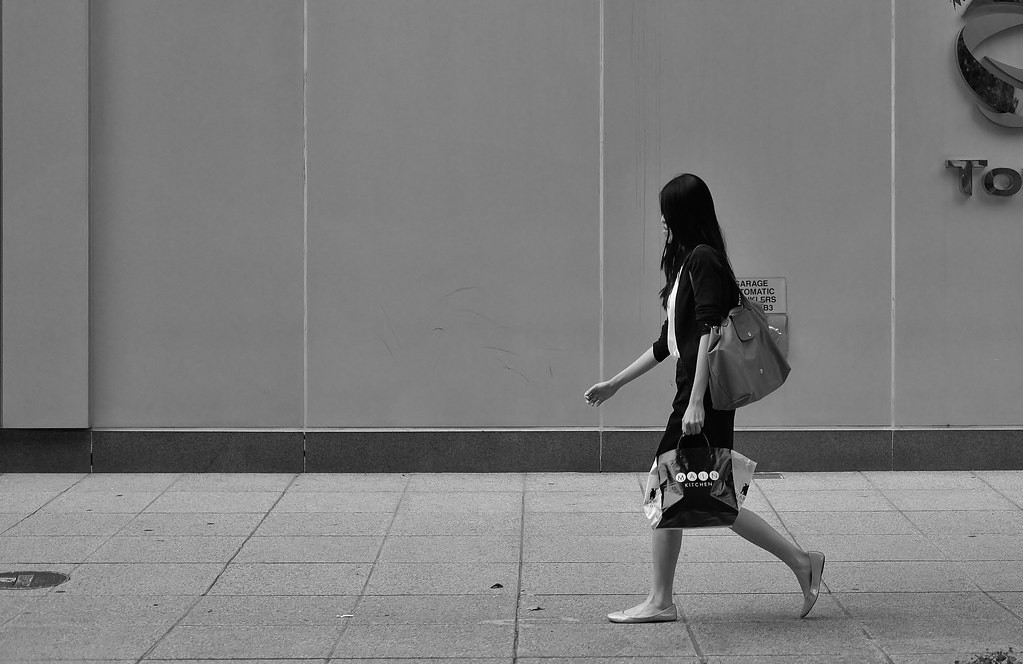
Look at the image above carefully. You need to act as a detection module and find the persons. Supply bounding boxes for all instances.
[581,174,827,623]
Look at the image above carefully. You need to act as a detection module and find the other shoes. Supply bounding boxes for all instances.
[606,603,677,622]
[800,551,825,618]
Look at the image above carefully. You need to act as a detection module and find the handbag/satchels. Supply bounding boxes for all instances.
[689,244,791,410]
[643,431,757,531]
